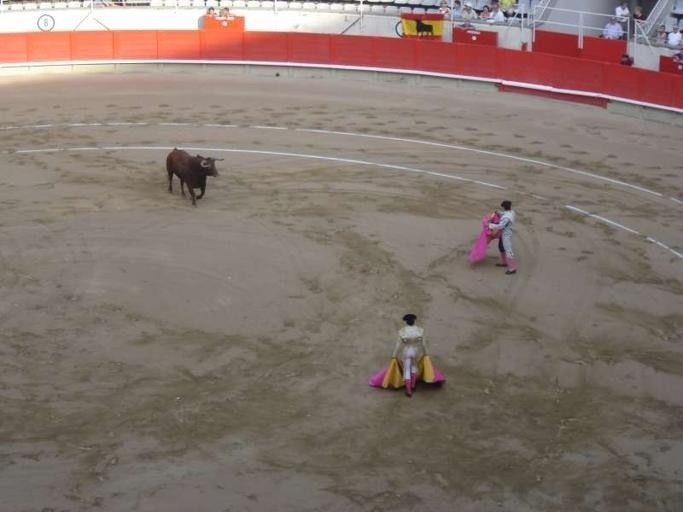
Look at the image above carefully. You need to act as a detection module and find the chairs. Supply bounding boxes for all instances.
[658,0,682,32]
[0,0,527,27]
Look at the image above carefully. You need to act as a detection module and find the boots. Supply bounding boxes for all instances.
[405,373,416,396]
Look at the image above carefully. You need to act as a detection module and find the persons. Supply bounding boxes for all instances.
[668,24,683,49]
[207,6,232,25]
[617,2,630,40]
[437,0,516,22]
[489,200,517,274]
[603,16,623,40]
[393,314,429,396]
[652,25,666,46]
[634,6,646,21]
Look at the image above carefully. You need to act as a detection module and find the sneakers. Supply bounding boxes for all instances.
[496,264,507,266]
[506,270,516,274]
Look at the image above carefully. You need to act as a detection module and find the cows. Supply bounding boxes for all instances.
[167,147,224,205]
